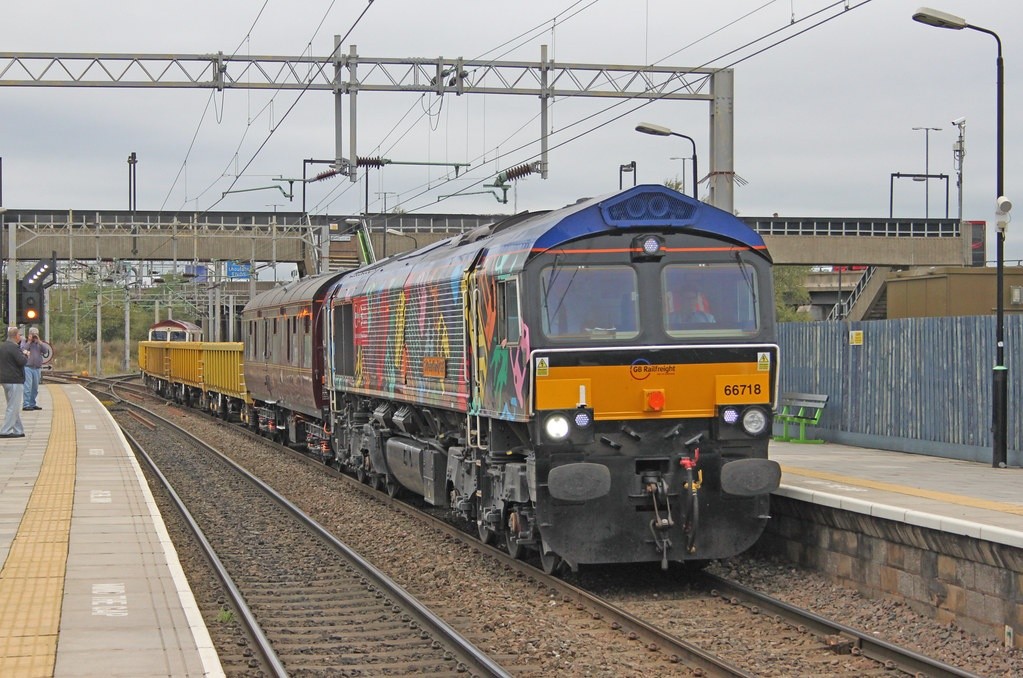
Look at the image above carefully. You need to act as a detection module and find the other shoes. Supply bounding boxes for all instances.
[0,434,25,438]
[22,405,42,411]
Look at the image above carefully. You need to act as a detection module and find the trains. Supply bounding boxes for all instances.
[149,320,203,343]
[137,184,783,575]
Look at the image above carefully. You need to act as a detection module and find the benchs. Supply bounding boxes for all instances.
[771,391,829,444]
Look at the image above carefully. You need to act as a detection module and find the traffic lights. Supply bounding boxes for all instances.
[21,292,39,323]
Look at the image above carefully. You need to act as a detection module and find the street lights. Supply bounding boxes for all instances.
[912,7,1009,468]
[635,121,698,200]
[912,127,943,218]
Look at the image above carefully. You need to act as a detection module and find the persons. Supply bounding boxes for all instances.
[0,327,30,437]
[22,327,48,411]
[545,275,583,334]
[668,284,716,325]
